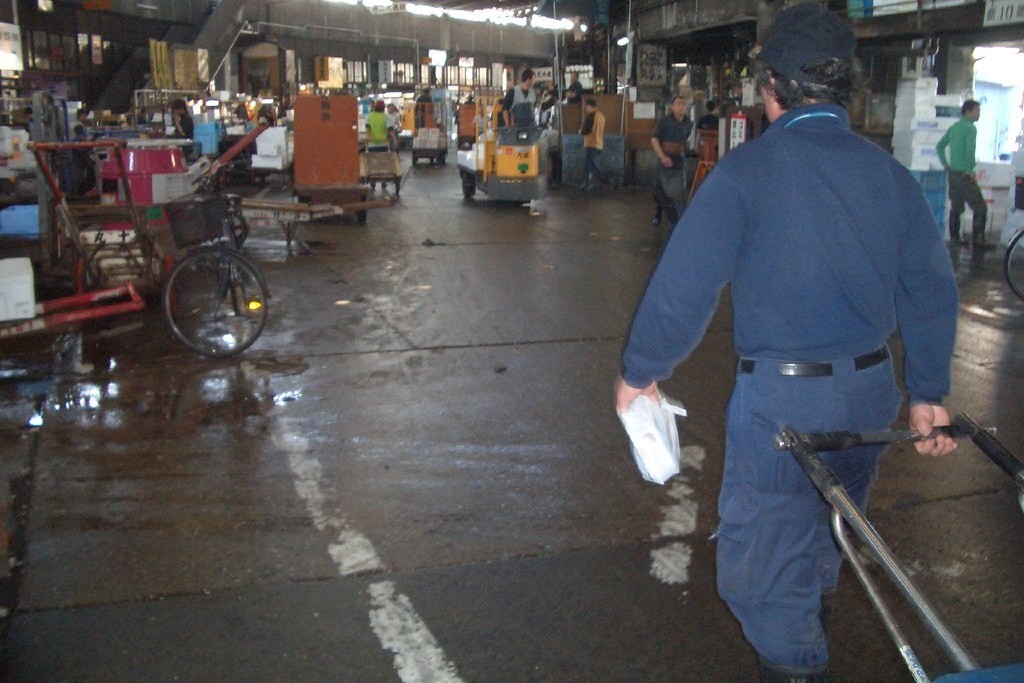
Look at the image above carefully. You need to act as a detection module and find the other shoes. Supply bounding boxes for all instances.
[652,217,661,225]
[759,655,829,683]
[819,585,838,629]
[950,235,969,247]
[973,233,998,249]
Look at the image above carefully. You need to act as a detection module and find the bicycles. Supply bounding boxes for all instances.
[160,160,272,361]
[1002,227,1024,302]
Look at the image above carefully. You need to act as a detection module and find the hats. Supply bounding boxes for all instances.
[373,100,384,108]
[747,4,858,87]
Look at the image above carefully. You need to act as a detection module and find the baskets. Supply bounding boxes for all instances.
[164,194,226,248]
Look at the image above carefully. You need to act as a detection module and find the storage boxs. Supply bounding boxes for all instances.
[457,143,484,170]
[252,127,288,169]
[413,128,446,149]
[0,257,35,321]
[891,77,964,237]
[964,163,1011,233]
[0,205,39,234]
[0,126,37,178]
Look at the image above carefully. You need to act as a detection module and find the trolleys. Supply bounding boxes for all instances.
[28,137,183,310]
[413,102,448,168]
[457,103,484,150]
[772,410,1024,683]
[362,138,402,196]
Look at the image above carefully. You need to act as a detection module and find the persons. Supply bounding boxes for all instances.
[615,5,957,683]
[937,100,998,250]
[235,70,719,226]
[25,100,197,159]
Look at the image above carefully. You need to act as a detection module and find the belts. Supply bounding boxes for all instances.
[740,346,888,376]
